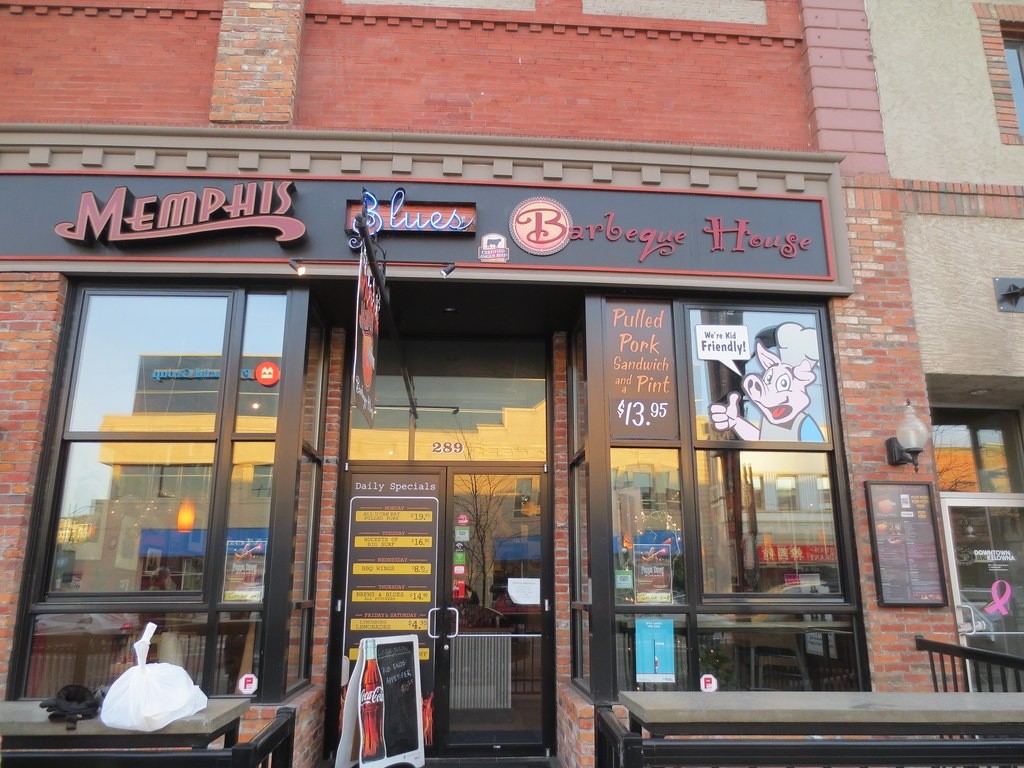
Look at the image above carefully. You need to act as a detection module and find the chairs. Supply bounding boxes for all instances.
[734,614,813,692]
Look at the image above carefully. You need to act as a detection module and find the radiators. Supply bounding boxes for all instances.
[449,627,515,709]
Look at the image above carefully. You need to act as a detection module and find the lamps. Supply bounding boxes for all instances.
[884,398,929,474]
[177,496,196,533]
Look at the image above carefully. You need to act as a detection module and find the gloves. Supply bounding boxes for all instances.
[38,683,110,731]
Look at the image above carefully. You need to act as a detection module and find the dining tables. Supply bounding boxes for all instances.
[128,619,263,700]
[619,692,1024,768]
[25,627,171,698]
[0,699,250,768]
[615,619,852,692]
